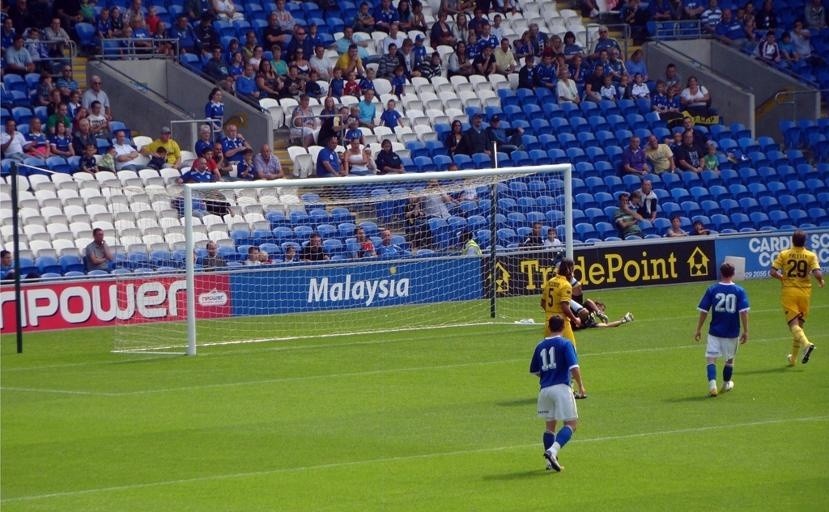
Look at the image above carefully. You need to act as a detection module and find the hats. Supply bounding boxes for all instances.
[471,113,506,120]
[160,127,171,134]
[416,33,426,41]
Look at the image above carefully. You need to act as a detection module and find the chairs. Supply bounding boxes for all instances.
[1,1,829,283]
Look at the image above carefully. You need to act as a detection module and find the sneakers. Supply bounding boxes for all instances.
[720,381,734,393]
[543,448,564,471]
[709,380,717,397]
[787,354,796,366]
[801,343,815,364]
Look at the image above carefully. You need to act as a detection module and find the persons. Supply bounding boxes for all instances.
[694,262,750,395]
[556,262,634,329]
[541,260,588,398]
[529,315,586,473]
[770,232,824,366]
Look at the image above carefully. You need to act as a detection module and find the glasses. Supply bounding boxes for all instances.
[454,123,462,128]
[60,66,103,86]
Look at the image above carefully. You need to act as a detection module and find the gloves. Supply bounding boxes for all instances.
[622,312,634,323]
[596,310,608,324]
